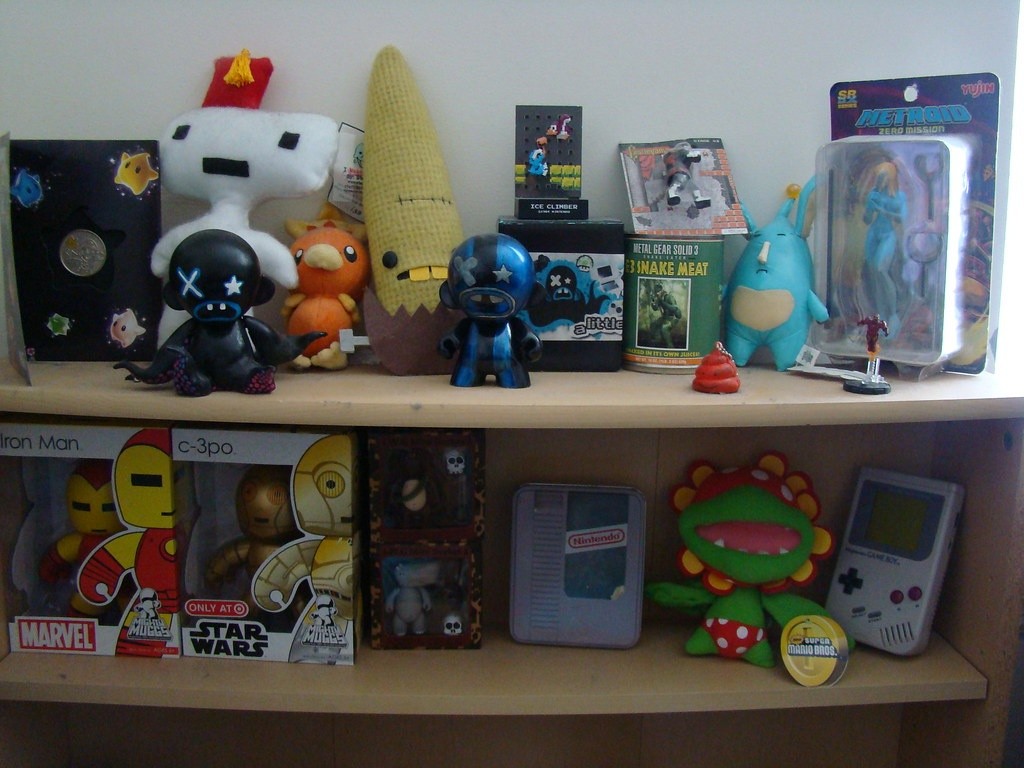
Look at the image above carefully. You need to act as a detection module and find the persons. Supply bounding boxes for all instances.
[204,464,318,635]
[39,459,141,626]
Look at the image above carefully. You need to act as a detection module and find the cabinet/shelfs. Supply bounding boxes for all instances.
[0,349,1024,718]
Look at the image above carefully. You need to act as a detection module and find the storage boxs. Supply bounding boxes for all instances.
[0,425,488,668]
[495,214,626,375]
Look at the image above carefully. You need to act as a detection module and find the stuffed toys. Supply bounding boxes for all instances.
[148,47,340,348]
[716,176,831,371]
[278,200,368,372]
[358,43,468,377]
[643,453,856,670]
